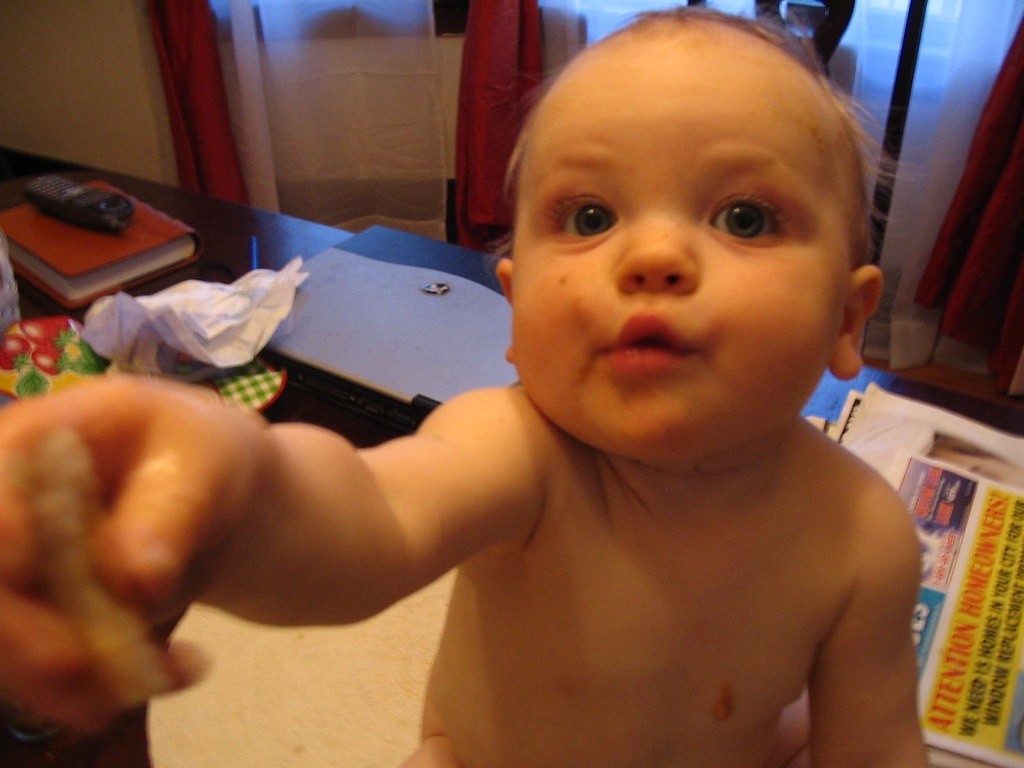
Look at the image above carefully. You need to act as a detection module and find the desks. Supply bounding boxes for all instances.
[0,146,1024,768]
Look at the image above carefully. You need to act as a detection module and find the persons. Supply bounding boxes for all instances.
[0,5,939,768]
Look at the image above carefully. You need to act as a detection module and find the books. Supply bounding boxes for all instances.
[0,180,203,311]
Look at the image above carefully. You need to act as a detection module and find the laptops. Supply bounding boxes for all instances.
[262,223,526,434]
